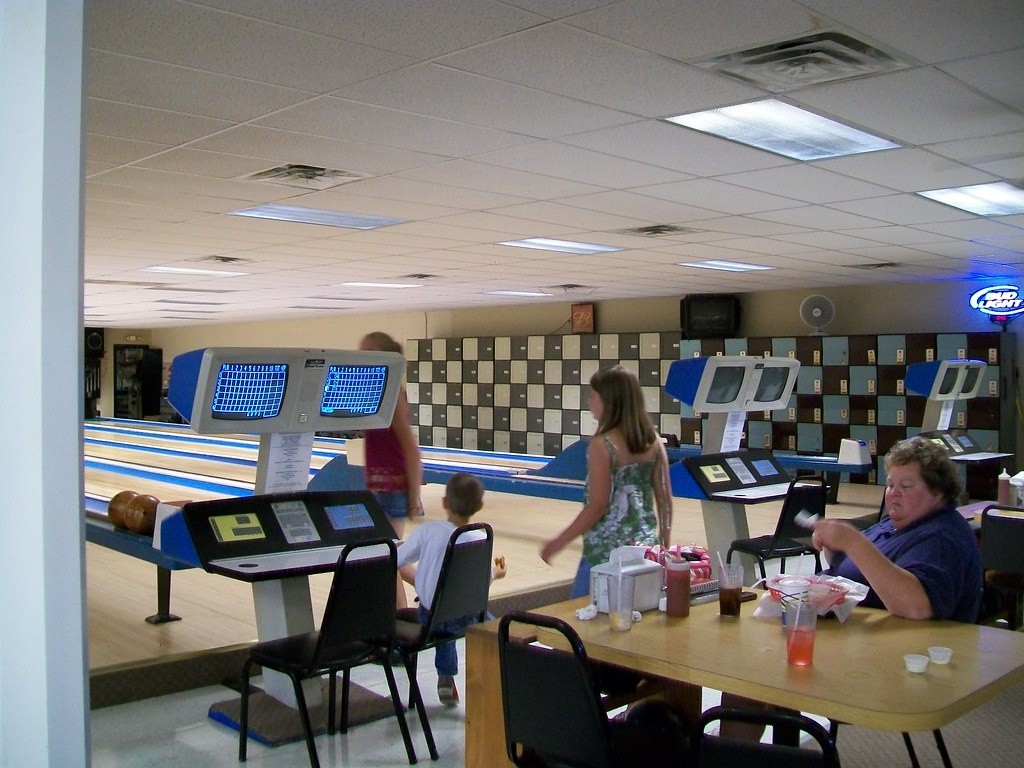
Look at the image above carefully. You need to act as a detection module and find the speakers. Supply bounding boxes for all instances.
[84,327,104,359]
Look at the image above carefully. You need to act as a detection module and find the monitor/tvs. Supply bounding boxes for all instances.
[961,368,979,393]
[939,368,958,394]
[680,294,741,340]
[212,363,289,420]
[706,366,745,405]
[320,365,388,418]
[754,367,789,401]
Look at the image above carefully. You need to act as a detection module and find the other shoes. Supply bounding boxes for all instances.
[437,676,460,705]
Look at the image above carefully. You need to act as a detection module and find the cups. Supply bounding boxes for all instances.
[606,576,635,632]
[785,602,817,667]
[778,578,811,630]
[904,654,929,673]
[718,564,745,618]
[928,647,954,665]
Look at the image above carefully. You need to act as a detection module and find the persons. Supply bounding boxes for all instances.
[396,472,505,709]
[720,434,983,742]
[358,331,423,667]
[539,362,673,603]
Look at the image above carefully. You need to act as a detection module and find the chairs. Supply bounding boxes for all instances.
[979,504,1024,632]
[341,522,494,760]
[686,706,841,768]
[498,611,689,768]
[726,475,826,590]
[239,537,418,768]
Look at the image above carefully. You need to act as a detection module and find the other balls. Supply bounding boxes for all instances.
[124,494,160,536]
[108,490,140,529]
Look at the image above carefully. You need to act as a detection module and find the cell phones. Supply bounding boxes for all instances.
[740,592,757,601]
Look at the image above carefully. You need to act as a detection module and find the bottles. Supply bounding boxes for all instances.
[998,468,1010,506]
[666,545,690,617]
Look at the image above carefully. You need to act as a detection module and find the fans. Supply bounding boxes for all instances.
[800,294,835,336]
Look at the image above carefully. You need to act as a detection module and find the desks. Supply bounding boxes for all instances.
[537,587,1024,768]
